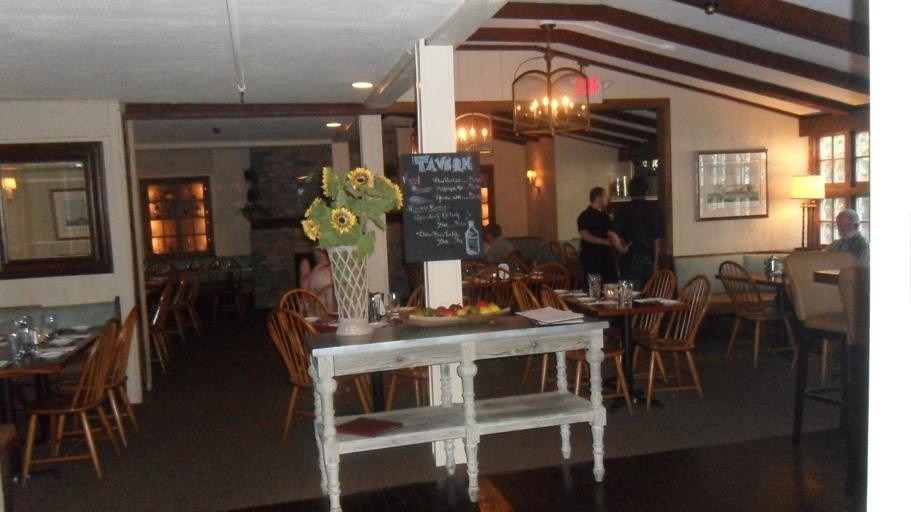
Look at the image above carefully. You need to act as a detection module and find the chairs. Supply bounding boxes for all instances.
[278,287,371,386]
[148,278,174,373]
[631,274,713,412]
[539,283,635,417]
[166,275,188,343]
[838,262,869,349]
[314,282,334,316]
[526,261,569,301]
[181,263,205,337]
[509,277,549,393]
[385,281,429,411]
[265,308,373,444]
[610,268,677,394]
[207,256,247,324]
[56,304,138,448]
[562,241,579,264]
[783,248,858,445]
[718,260,796,369]
[467,266,515,310]
[548,240,562,260]
[21,317,120,482]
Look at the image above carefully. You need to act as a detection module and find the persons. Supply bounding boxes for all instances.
[484,222,514,262]
[827,210,870,266]
[608,212,665,291]
[577,187,613,285]
[306,266,335,314]
[299,244,329,314]
[614,179,665,265]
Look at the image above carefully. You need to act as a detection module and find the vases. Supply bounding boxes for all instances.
[326,245,374,336]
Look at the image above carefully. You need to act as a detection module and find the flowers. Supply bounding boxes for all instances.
[300,164,404,258]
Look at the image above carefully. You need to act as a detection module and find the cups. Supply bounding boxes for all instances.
[603,284,614,304]
[9,332,31,366]
[619,280,633,309]
[588,274,600,299]
[387,293,400,315]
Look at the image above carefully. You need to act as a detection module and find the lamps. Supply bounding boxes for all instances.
[455,110,494,155]
[227,0,246,92]
[2,175,18,201]
[790,174,826,250]
[526,170,540,189]
[511,25,591,139]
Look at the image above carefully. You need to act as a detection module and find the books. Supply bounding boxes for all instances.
[337,417,402,438]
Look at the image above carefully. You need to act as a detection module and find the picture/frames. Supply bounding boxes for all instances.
[694,148,769,223]
[49,187,90,241]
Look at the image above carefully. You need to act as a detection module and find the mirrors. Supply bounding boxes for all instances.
[0,140,114,281]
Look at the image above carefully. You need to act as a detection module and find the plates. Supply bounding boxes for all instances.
[409,315,458,322]
[578,297,596,303]
[659,299,679,305]
[40,347,64,360]
[50,339,73,346]
[554,290,568,294]
[575,293,587,297]
[461,306,512,325]
[71,324,93,332]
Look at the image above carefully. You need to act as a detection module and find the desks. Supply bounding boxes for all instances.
[300,315,609,512]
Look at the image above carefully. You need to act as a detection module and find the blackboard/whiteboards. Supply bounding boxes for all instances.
[398,149,486,264]
